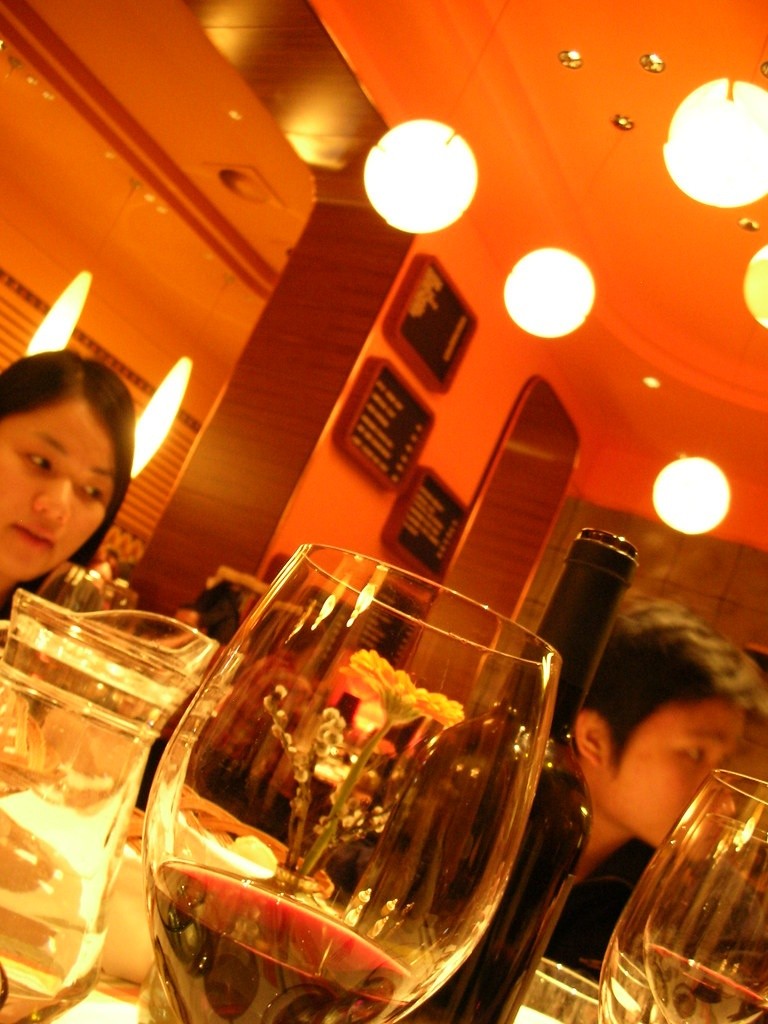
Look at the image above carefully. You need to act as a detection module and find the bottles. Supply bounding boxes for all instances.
[303,527,640,1022]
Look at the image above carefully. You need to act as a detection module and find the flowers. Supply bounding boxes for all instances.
[258,644,476,894]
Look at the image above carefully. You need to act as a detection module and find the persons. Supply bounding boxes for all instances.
[170,581,330,807]
[325,593,761,983]
[0,351,134,612]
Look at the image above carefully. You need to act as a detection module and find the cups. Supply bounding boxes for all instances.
[0,586,215,1023]
[598,767,767,1024]
[136,542,567,1024]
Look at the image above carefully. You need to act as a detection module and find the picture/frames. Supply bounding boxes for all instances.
[382,465,468,584]
[332,357,435,493]
[382,252,478,393]
